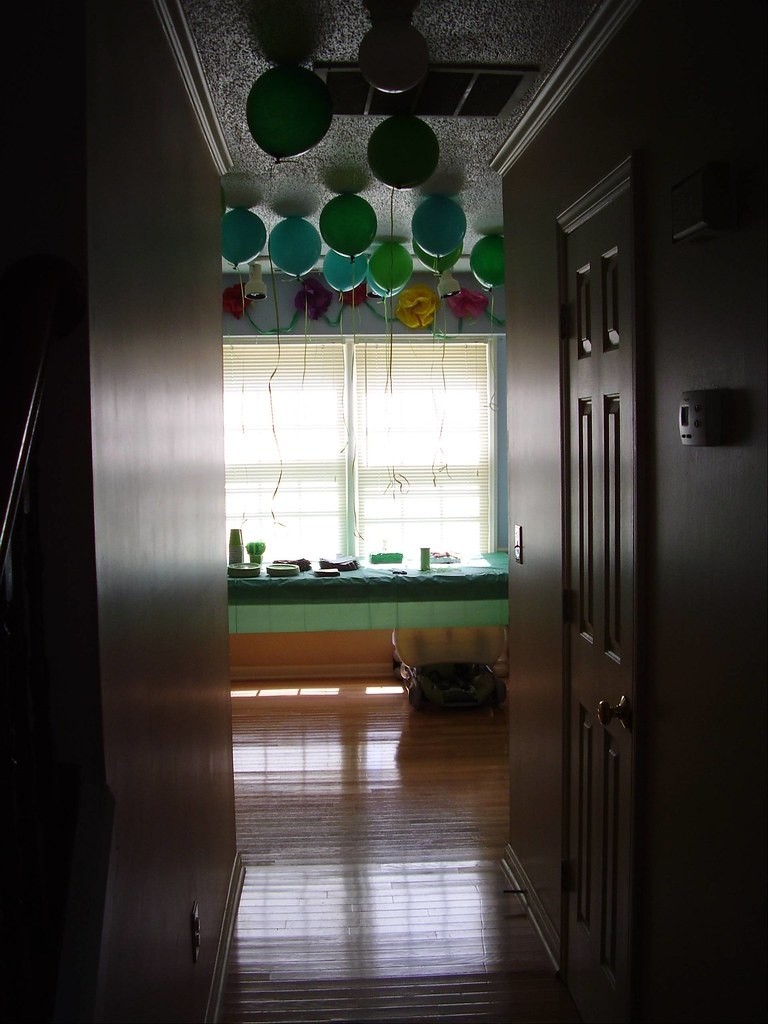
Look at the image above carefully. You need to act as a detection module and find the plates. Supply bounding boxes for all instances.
[266,563,300,576]
[228,562,259,578]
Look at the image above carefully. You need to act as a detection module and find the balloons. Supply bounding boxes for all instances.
[246,65,332,163]
[319,194,467,302]
[221,209,266,269]
[470,234,507,291]
[367,115,439,189]
[268,217,321,281]
[359,22,429,93]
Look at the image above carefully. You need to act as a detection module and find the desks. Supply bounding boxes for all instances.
[228,552,509,633]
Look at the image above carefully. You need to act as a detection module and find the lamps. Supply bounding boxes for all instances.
[366,283,382,299]
[437,272,461,299]
[245,261,267,300]
